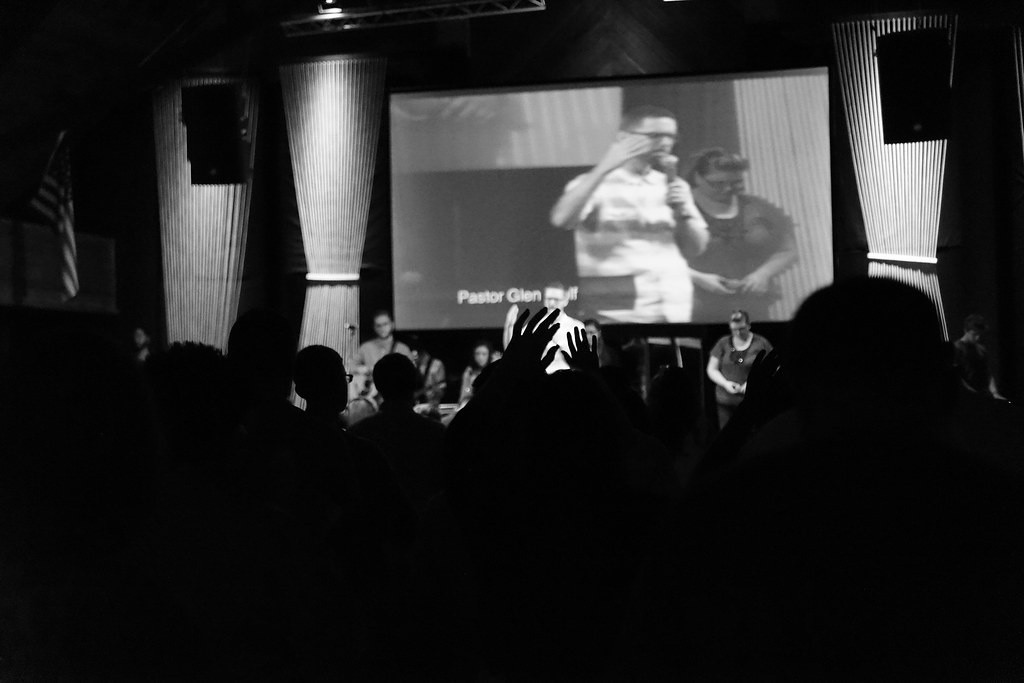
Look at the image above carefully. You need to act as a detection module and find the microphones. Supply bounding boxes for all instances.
[343,323,359,330]
[661,154,679,209]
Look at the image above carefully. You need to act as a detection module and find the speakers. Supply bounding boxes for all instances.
[875,27,953,143]
[184,82,248,184]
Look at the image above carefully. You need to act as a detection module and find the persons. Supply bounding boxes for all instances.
[0,276,1024,683]
[353,308,417,400]
[706,310,775,426]
[583,319,609,367]
[684,149,799,322]
[549,106,709,323]
[951,313,1010,400]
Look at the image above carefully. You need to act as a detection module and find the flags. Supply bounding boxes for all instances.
[39,141,80,298]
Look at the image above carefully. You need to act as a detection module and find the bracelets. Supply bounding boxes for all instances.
[674,214,691,221]
[992,391,998,395]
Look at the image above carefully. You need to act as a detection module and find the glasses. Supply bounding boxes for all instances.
[629,130,679,142]
[703,174,745,191]
[343,374,353,382]
